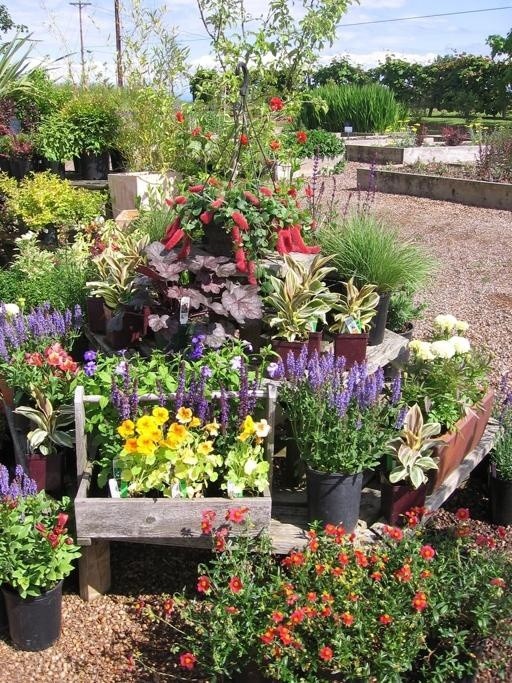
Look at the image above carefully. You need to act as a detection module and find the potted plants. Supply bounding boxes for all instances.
[320,212,433,346]
[0,90,134,179]
[107,0,186,221]
[377,401,447,527]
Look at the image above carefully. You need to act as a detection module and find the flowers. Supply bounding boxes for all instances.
[491,369,511,480]
[1,178,394,598]
[400,312,497,428]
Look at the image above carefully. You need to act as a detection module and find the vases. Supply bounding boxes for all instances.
[487,472,512,527]
[388,391,494,495]
[2,581,64,652]
